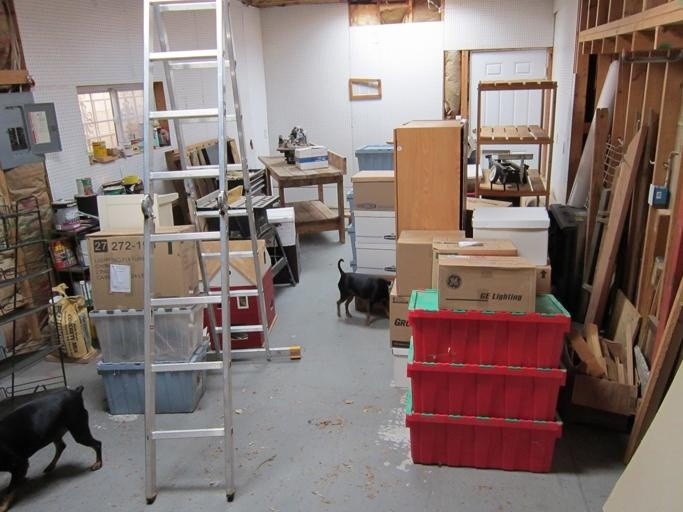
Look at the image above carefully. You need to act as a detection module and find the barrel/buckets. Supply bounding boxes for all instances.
[52,199,80,231]
[49,237,78,269]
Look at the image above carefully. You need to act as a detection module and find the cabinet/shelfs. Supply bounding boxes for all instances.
[50,216,100,308]
[393,120,470,240]
[475,79,557,211]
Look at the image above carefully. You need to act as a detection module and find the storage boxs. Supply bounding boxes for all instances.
[88,292,208,363]
[388,277,411,350]
[535,264,552,293]
[431,238,518,290]
[404,385,564,474]
[197,239,273,289]
[406,335,568,421]
[96,336,211,414]
[294,146,329,170]
[86,224,199,310]
[197,266,276,348]
[396,229,468,297]
[437,254,537,313]
[96,193,180,230]
[408,288,572,369]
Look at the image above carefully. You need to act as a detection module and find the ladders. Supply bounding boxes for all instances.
[143,0,273,505]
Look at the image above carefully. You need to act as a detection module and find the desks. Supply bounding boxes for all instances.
[258,155,347,243]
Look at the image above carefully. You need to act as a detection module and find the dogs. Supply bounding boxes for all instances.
[0,385,102,512]
[336,258,394,326]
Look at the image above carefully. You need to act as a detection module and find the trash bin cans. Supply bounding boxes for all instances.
[549,203,601,321]
